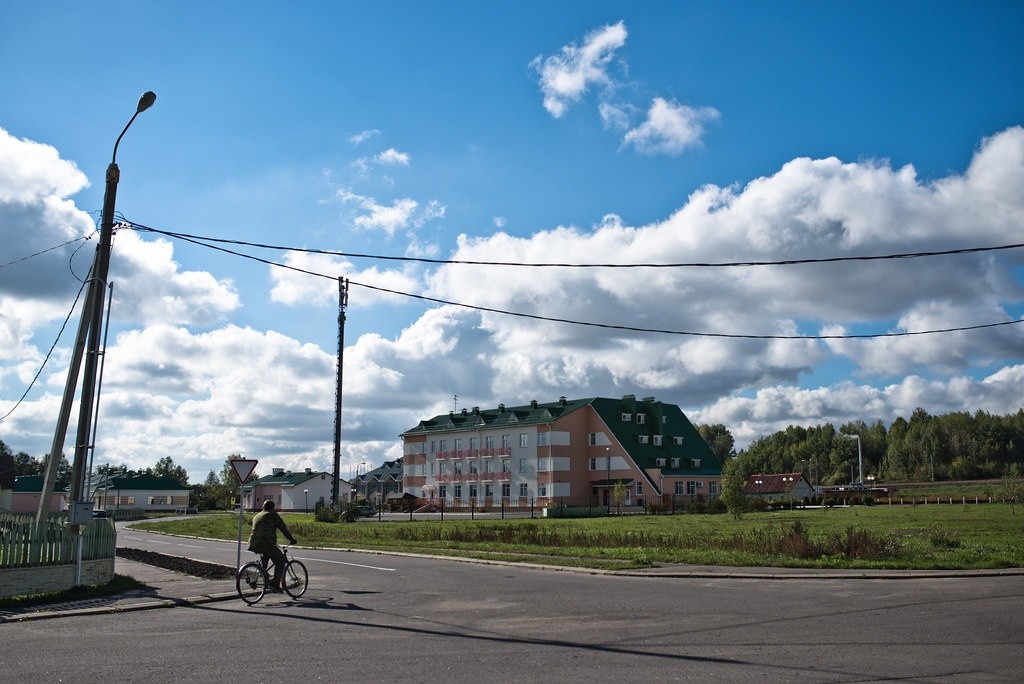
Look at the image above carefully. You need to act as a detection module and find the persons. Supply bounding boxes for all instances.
[248,501,298,595]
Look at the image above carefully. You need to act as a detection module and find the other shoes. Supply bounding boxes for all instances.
[268,582,284,594]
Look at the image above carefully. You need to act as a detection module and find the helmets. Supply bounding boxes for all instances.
[263,500,275,510]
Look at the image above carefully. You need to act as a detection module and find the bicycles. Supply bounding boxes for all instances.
[235,540,309,604]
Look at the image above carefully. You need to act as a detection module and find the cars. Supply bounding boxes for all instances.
[341,505,377,517]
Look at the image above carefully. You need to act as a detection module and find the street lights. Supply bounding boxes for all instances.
[782,476,794,510]
[868,474,876,488]
[845,462,854,483]
[606,448,611,517]
[755,477,762,495]
[65,92,158,560]
[356,463,365,501]
[802,458,812,487]
